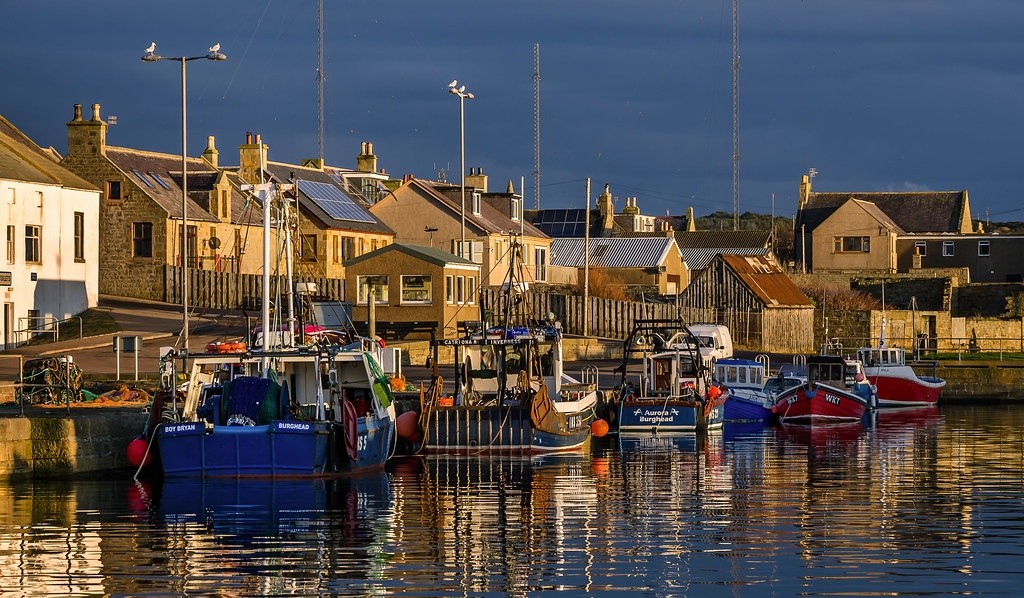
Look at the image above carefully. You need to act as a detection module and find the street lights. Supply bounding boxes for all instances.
[450,88,474,259]
[140,52,227,373]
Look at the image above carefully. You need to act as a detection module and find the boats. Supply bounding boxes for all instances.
[608,313,730,435]
[844,355,878,407]
[141,140,398,478]
[762,363,809,396]
[770,317,868,428]
[417,229,598,457]
[713,358,776,420]
[856,279,947,409]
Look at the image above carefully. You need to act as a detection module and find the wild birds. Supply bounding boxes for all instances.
[144,42,157,55]
[206,42,221,53]
[448,79,458,88]
[458,85,466,93]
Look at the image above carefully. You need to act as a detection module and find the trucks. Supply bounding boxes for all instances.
[688,324,733,374]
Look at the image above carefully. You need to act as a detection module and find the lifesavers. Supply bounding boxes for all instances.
[205,340,247,354]
